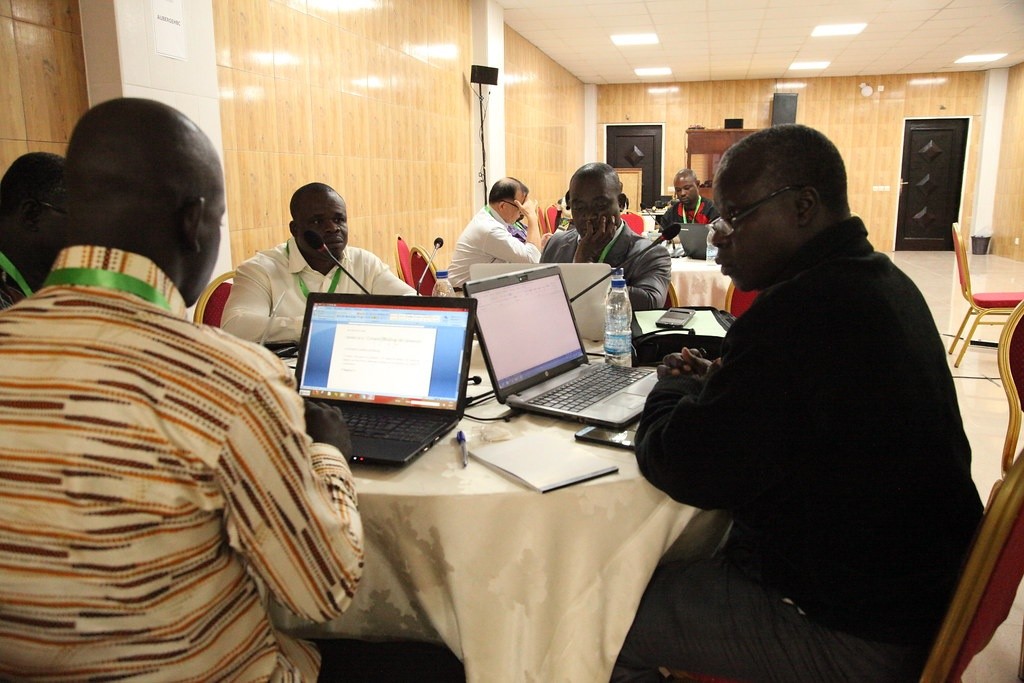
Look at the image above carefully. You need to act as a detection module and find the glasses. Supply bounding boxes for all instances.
[712,185,803,237]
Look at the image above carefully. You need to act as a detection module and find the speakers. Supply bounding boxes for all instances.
[470,65,498,85]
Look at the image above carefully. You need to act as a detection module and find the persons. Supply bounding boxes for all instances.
[609,124,985,683]
[659,169,720,232]
[0,151,67,311]
[539,162,672,310]
[220,182,423,346]
[0,99,465,683]
[447,176,555,292]
[558,189,630,225]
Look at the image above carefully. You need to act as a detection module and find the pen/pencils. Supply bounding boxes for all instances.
[457,431,467,466]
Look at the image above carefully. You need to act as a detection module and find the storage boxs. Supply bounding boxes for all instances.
[724,119,744,129]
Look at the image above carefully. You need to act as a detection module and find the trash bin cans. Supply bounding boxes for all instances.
[970,236,991,255]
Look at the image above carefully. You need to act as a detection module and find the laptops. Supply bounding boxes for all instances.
[462,263,661,430]
[294,292,478,467]
[680,223,709,260]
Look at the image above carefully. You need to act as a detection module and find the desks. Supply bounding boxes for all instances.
[671,256,731,312]
[284,338,699,683]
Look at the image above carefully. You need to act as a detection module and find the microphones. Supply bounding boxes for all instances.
[570,223,681,303]
[639,203,663,231]
[303,231,370,295]
[416,238,443,296]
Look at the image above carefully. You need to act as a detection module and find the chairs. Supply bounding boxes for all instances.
[408,245,437,297]
[948,221,1024,369]
[534,206,548,238]
[998,299,1024,476]
[921,446,1024,683]
[544,204,558,234]
[620,209,647,236]
[664,281,679,308]
[725,279,762,319]
[393,233,413,289]
[191,270,235,328]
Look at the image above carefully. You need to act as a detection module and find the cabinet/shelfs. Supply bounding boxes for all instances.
[686,129,760,200]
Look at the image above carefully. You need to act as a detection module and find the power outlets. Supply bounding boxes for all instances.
[1015,237,1020,245]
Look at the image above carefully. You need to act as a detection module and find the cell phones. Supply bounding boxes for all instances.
[574,426,636,450]
[656,307,695,328]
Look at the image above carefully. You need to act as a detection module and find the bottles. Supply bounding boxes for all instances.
[707,225,719,265]
[432,271,457,298]
[651,231,659,246]
[604,279,632,367]
[607,268,629,303]
[652,205,656,213]
